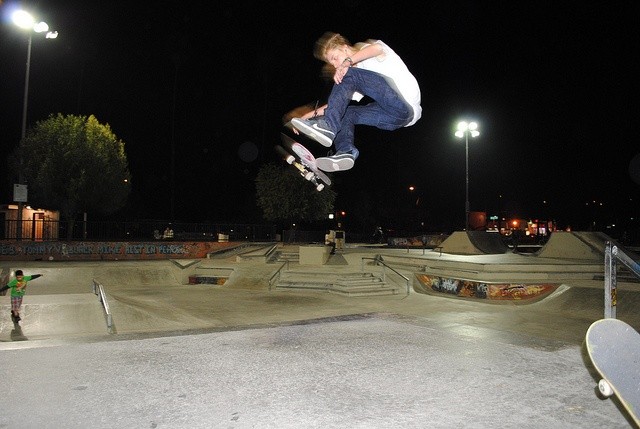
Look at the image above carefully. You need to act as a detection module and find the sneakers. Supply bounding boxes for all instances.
[315,153,355,172]
[291,117,336,147]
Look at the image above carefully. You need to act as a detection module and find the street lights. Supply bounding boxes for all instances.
[12,9,59,242]
[454,121,480,230]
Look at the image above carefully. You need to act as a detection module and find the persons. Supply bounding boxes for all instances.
[0,270,44,319]
[290,31,424,173]
[324,229,336,255]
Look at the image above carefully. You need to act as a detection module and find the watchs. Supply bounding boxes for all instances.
[344,56,354,68]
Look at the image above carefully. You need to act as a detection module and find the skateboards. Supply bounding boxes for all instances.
[585,319,640,428]
[287,143,331,191]
[11,310,21,323]
